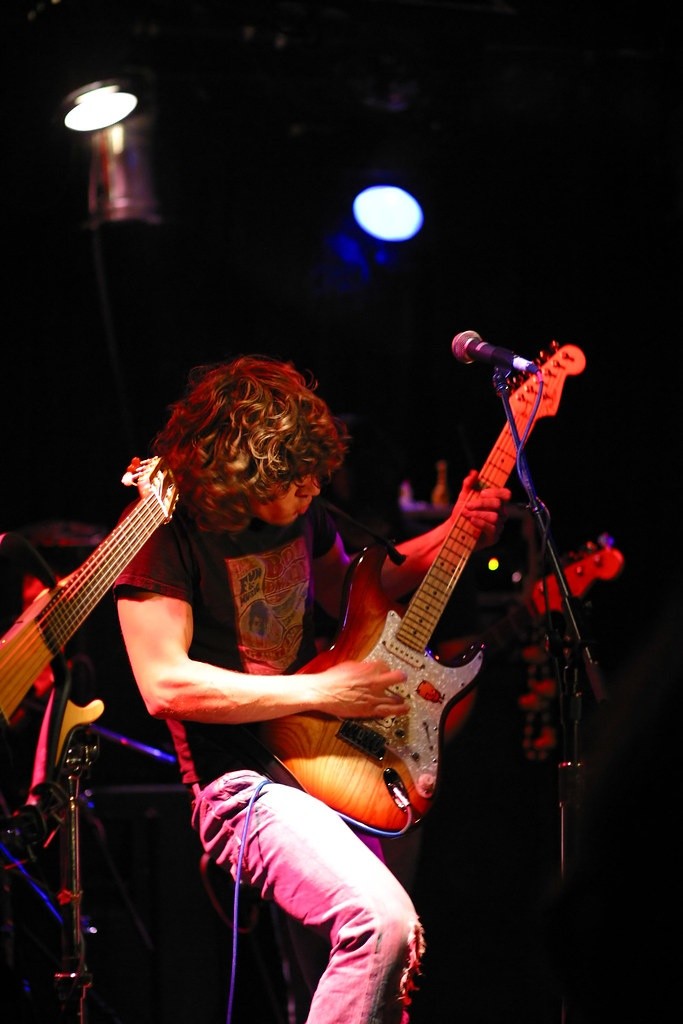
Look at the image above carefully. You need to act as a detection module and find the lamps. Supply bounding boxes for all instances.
[62,58,137,133]
[353,185,422,241]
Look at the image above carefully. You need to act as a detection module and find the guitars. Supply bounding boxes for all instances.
[426,530,627,749]
[259,337,589,842]
[0,454,183,880]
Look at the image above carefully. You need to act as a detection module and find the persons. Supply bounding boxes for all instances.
[107,355,514,1024]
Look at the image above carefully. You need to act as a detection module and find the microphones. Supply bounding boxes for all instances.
[452,330,540,374]
